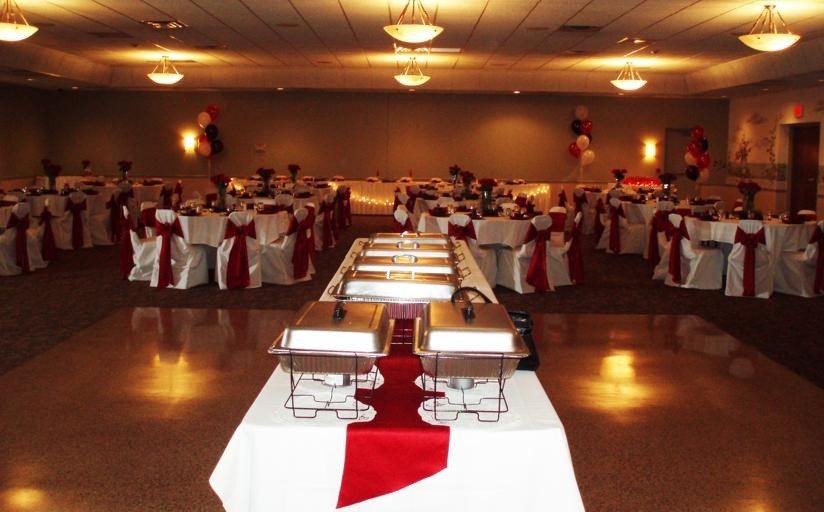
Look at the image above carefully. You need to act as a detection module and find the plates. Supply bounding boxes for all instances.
[415,178,532,219]
[581,177,793,224]
[171,174,335,215]
[19,173,162,196]
[332,175,410,183]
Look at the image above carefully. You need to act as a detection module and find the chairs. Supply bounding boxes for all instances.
[1,158,184,285]
[393,165,586,298]
[562,166,824,300]
[116,162,351,293]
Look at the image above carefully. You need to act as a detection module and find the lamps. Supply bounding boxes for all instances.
[384,0,444,45]
[610,59,647,91]
[145,55,185,85]
[0,0,39,42]
[393,56,431,87]
[738,4,801,53]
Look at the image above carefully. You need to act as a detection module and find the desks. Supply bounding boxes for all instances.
[330,180,551,232]
[208,236,588,511]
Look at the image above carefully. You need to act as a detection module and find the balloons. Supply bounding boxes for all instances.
[195,103,223,157]
[568,104,595,166]
[682,124,710,184]
